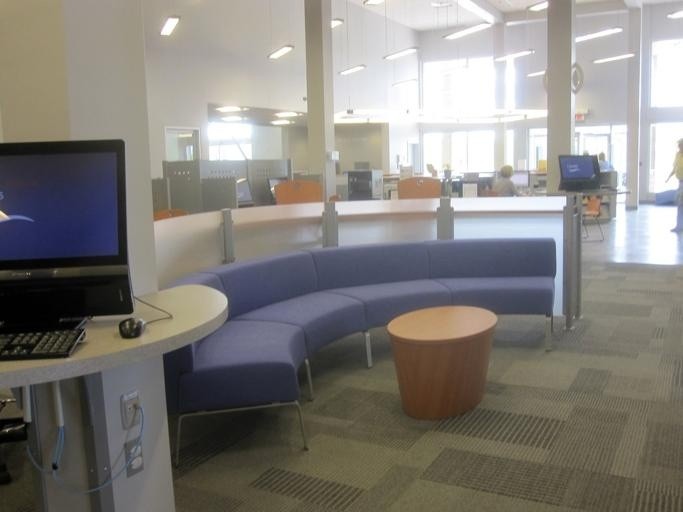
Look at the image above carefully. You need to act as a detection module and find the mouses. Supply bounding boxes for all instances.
[119,317,146,338]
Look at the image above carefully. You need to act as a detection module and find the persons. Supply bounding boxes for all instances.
[492,165,524,197]
[599,153,610,172]
[665,138,683,233]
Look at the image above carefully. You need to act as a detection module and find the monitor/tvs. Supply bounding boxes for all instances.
[1,139,134,318]
[557,155,600,191]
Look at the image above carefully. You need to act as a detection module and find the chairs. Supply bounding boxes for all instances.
[480,190,497,196]
[274,180,323,204]
[582,196,605,240]
[398,176,441,199]
[153,209,189,220]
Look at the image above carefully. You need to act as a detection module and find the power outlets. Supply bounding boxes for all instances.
[124,438,144,478]
[49,459,77,481]
[71,416,91,438]
[120,390,142,430]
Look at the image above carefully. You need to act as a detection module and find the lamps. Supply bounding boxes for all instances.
[594,54,635,67]
[440,0,490,40]
[494,24,534,60]
[161,16,180,37]
[574,0,622,42]
[381,0,419,60]
[527,11,545,76]
[339,0,365,76]
[264,0,293,59]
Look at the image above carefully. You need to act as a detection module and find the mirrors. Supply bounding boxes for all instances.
[204,102,310,205]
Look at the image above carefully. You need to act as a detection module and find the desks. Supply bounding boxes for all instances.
[529,172,616,223]
[0,283,229,512]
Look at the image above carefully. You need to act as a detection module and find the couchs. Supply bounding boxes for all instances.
[164,238,556,466]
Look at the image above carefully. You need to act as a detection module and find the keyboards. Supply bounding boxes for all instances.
[1,330,87,360]
